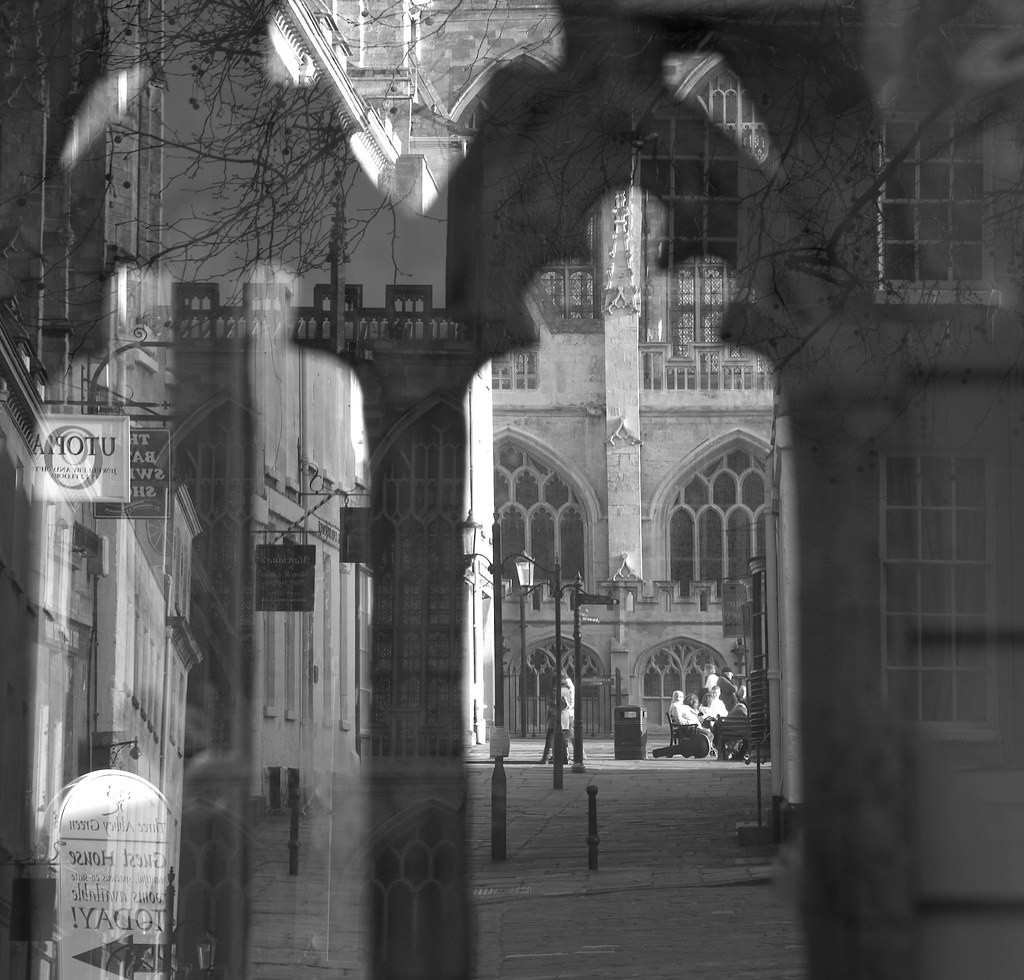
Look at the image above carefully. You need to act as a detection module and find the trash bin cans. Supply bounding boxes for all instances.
[614,704,648,759]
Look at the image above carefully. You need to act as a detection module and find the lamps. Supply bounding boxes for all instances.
[92,735,141,768]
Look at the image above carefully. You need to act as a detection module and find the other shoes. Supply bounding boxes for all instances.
[537,758,549,764]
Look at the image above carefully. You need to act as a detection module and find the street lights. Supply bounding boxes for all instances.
[463,508,564,862]
[514,546,613,788]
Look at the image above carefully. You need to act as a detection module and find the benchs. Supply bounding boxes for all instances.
[665,712,699,758]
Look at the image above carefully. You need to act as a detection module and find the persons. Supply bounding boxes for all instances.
[537,666,587,764]
[670,660,748,757]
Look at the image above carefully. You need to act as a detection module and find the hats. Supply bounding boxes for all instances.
[722,667,733,673]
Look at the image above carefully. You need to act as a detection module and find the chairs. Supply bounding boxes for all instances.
[716,714,752,761]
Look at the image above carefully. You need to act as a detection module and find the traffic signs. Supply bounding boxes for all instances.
[51,767,177,979]
